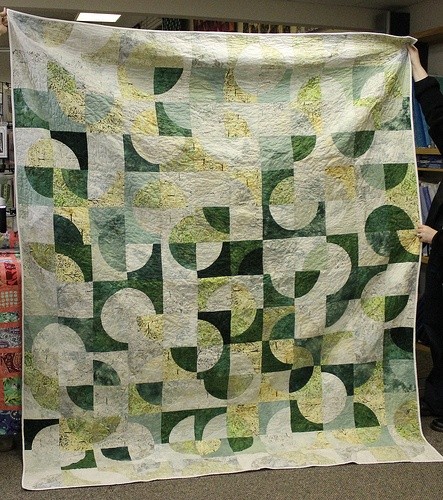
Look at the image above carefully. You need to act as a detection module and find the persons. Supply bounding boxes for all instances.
[403,43,443,432]
[0,7,8,35]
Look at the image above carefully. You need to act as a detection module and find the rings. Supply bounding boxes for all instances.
[417,233,420,237]
